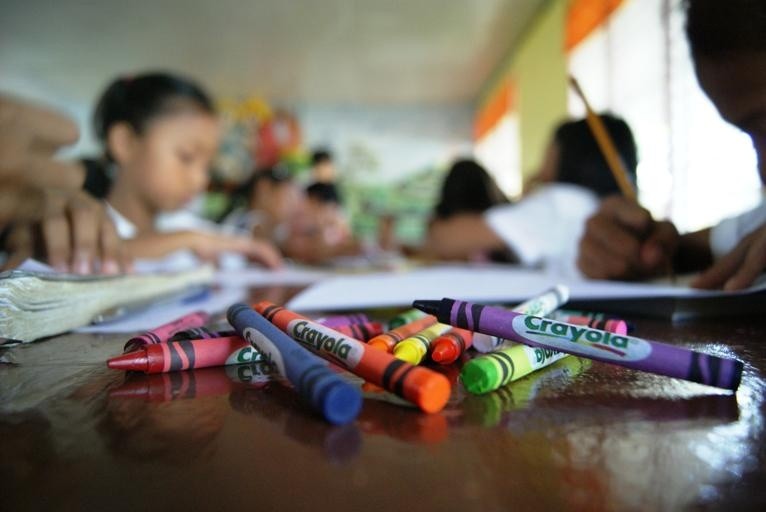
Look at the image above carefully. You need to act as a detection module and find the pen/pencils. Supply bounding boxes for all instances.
[568,76,676,286]
[413,297,744,398]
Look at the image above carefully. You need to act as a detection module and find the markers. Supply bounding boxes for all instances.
[106,286,626,425]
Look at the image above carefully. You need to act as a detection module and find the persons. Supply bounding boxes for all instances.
[2,69,641,279]
[571,1,766,295]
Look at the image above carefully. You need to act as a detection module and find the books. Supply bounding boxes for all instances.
[0,265,215,345]
